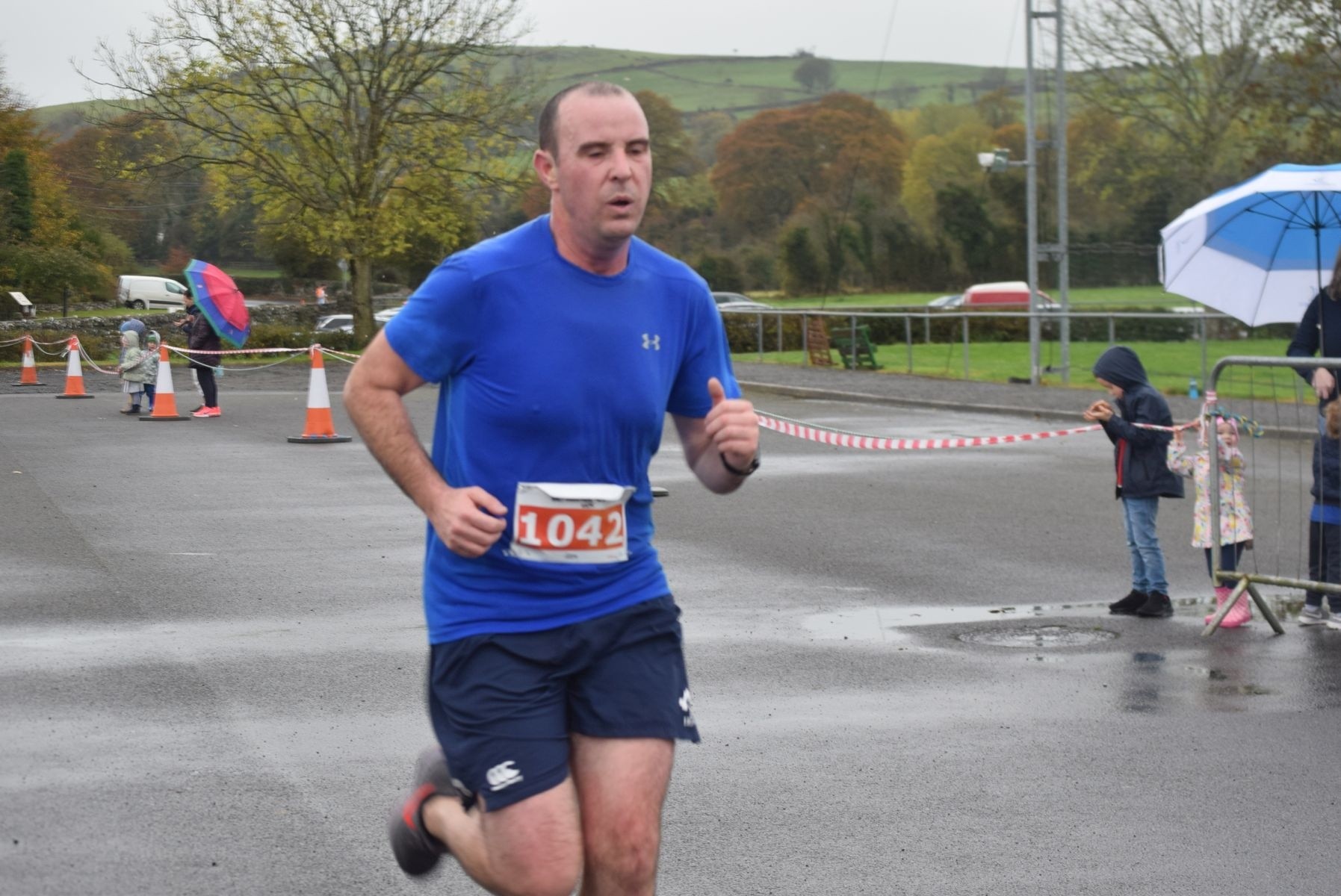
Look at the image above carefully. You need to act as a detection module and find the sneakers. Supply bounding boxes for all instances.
[1137,590,1174,616]
[190,405,221,417]
[1298,606,1341,629]
[1109,588,1147,612]
[391,751,465,877]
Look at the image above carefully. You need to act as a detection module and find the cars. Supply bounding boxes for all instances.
[711,292,768,310]
[928,282,1062,308]
[318,308,404,332]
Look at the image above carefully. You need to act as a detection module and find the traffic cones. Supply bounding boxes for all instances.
[15,335,46,386]
[59,333,94,399]
[139,343,192,422]
[286,349,353,445]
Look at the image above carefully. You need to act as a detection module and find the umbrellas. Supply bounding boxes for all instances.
[1157,159,1341,359]
[182,259,251,349]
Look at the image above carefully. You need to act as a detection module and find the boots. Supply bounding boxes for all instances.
[120,404,140,413]
[1205,586,1251,629]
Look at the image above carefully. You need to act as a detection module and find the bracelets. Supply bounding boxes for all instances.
[719,443,762,476]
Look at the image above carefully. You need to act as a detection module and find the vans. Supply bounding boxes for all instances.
[118,275,190,312]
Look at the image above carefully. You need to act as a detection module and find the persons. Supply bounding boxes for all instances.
[315,284,329,305]
[1282,240,1340,439]
[1167,412,1255,628]
[117,319,161,415]
[1081,345,1187,615]
[172,289,223,417]
[343,80,763,895]
[1297,397,1341,628]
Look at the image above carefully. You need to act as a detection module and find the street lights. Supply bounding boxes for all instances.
[977,147,1045,381]
